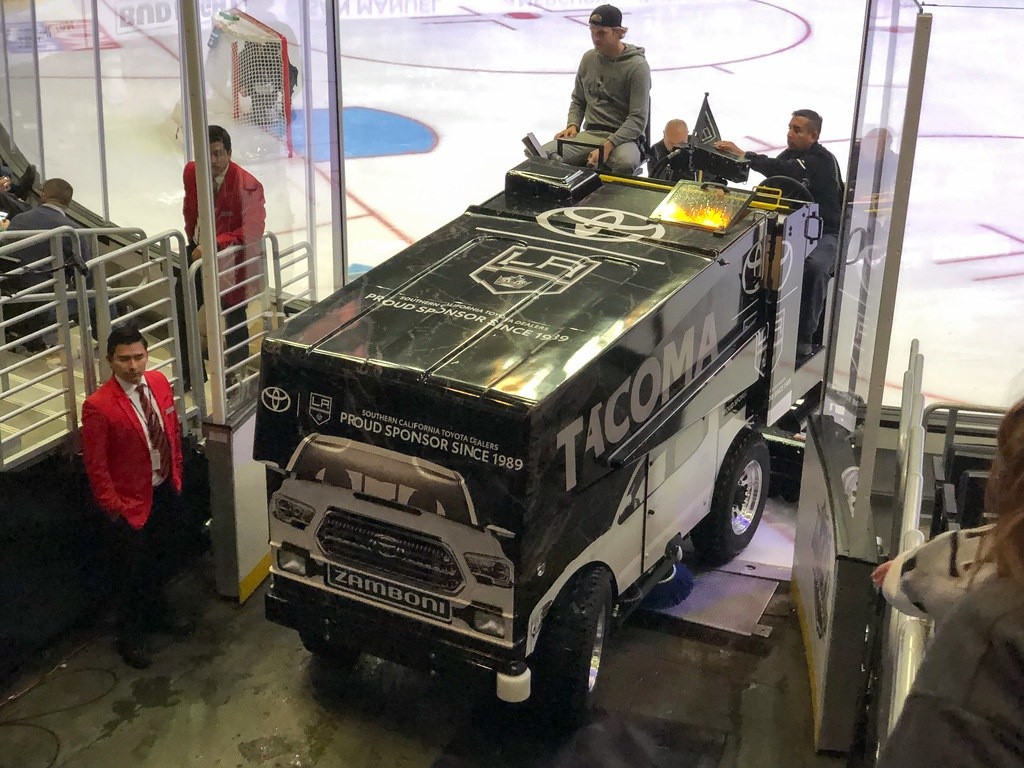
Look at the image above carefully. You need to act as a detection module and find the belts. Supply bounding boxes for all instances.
[153,482,170,496]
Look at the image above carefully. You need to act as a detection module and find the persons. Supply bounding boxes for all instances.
[648,119,728,187]
[713,109,844,354]
[171,125,266,398]
[0,164,122,347]
[852,127,901,265]
[541,4,651,179]
[82,326,196,671]
[870,399,1024,768]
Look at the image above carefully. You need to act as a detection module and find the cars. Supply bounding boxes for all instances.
[259,433,519,652]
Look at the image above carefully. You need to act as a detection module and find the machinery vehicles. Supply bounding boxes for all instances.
[250,133,851,719]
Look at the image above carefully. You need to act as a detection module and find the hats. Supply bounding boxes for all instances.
[589,4,623,27]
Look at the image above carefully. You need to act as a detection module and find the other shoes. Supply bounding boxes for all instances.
[797,343,813,355]
[144,605,195,635]
[183,369,208,392]
[111,634,151,668]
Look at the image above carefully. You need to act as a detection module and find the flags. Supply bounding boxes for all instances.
[692,99,723,148]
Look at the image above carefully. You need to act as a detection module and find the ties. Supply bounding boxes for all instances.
[136,385,172,478]
[213,181,217,194]
